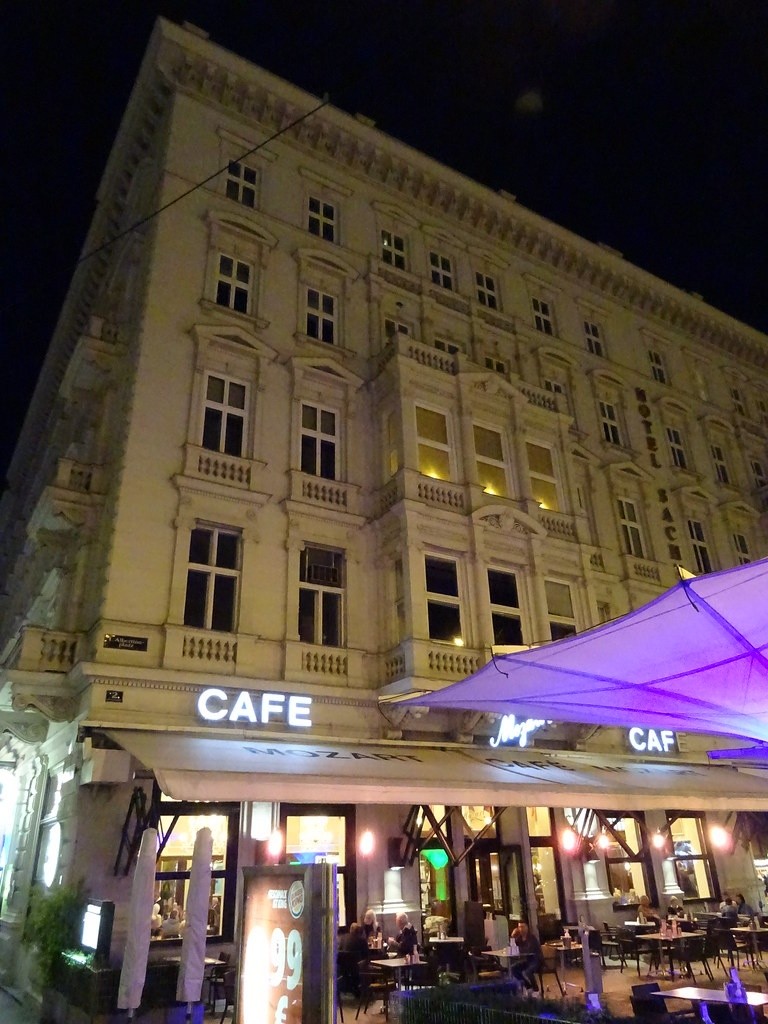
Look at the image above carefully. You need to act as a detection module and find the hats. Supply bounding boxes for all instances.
[364,909,375,925]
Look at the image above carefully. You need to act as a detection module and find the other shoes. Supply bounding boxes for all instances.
[531,991,539,997]
[525,988,533,997]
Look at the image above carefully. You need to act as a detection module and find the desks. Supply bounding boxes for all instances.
[624,920,656,958]
[163,953,226,1008]
[545,941,583,995]
[668,915,698,933]
[562,925,596,943]
[429,936,464,974]
[729,926,768,966]
[370,957,428,991]
[649,986,768,1024]
[481,949,536,981]
[635,930,702,977]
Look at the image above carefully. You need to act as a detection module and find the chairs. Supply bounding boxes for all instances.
[521,945,565,1001]
[205,952,230,1003]
[338,952,397,1022]
[616,925,657,977]
[603,922,628,962]
[599,931,628,967]
[669,930,713,985]
[627,994,674,1024]
[463,951,501,982]
[708,916,737,963]
[631,983,673,1024]
[208,965,236,1015]
[468,946,509,975]
[580,930,606,967]
[712,928,750,970]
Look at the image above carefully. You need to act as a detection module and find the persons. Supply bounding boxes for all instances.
[339,909,379,964]
[637,895,661,922]
[719,891,753,916]
[388,912,418,957]
[668,896,683,915]
[511,919,543,998]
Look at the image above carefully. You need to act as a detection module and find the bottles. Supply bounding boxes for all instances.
[413,945,419,963]
[377,933,382,948]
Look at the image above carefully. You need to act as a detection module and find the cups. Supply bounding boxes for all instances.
[372,939,378,948]
[406,955,410,963]
[410,955,415,963]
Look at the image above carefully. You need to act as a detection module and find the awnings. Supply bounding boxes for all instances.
[89,728,768,867]
